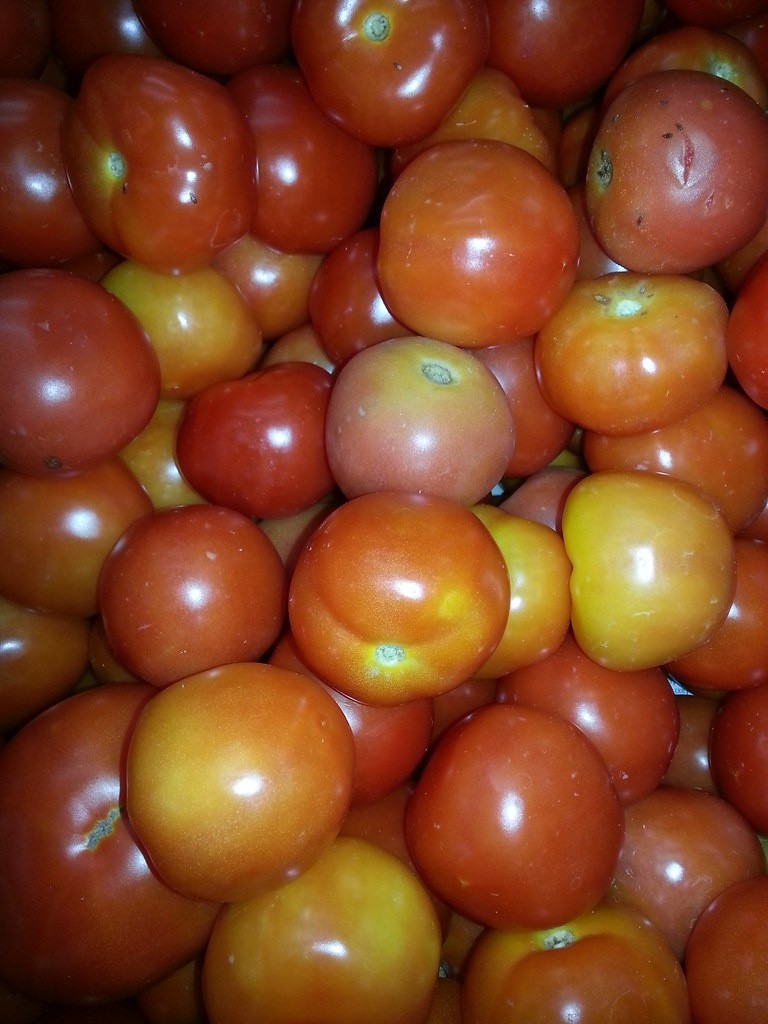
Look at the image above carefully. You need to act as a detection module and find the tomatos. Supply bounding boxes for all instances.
[0,0,768,1024]
